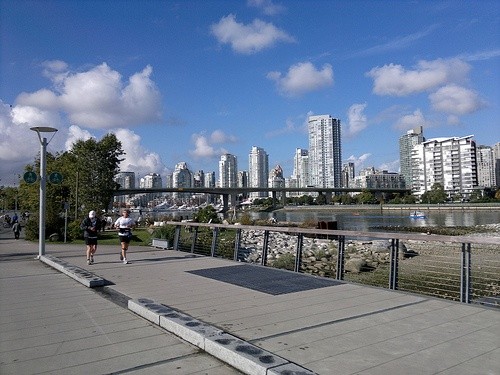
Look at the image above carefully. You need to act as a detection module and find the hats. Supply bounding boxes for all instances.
[88,210,95,219]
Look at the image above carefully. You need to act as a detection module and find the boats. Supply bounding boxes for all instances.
[410,213,426,218]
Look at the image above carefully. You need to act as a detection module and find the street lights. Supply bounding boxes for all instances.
[30,127,58,259]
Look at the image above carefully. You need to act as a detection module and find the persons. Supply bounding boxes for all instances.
[5,215,11,226]
[80,210,101,265]
[114,209,136,264]
[13,213,18,222]
[22,211,30,220]
[12,220,21,240]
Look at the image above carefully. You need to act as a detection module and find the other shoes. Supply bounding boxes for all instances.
[123,260,128,264]
[120,252,123,260]
[90,256,94,262]
[87,260,91,265]
